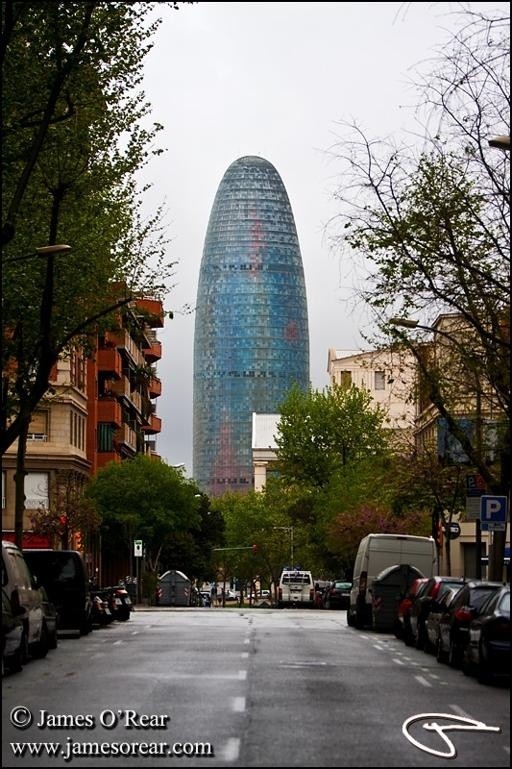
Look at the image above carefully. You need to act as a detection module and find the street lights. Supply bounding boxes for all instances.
[272,525,294,572]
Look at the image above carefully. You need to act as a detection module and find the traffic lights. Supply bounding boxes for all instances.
[74,531,86,554]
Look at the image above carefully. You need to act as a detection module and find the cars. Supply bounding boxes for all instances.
[345,532,512,691]
[199,564,353,610]
[1,538,135,678]
